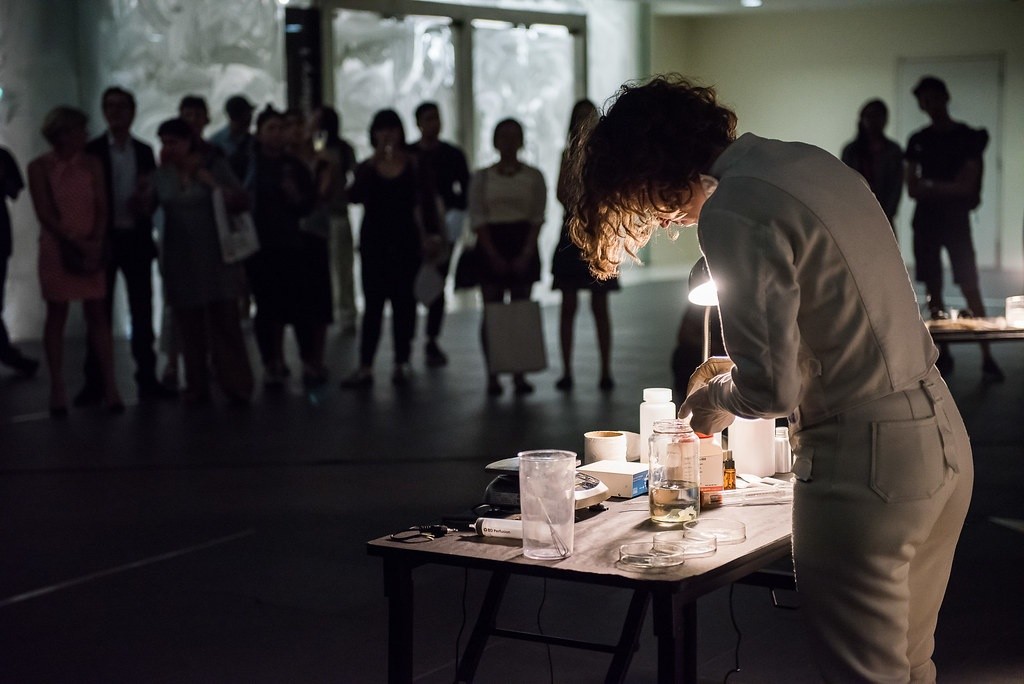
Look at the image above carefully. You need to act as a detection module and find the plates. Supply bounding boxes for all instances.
[619,518,747,569]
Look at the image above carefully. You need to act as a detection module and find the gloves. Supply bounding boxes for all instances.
[679,390,733,436]
[687,355,735,394]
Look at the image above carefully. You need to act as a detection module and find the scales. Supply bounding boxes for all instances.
[485,458,610,521]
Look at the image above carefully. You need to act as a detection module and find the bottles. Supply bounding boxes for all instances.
[649,421,701,523]
[639,388,676,465]
[775,426,792,473]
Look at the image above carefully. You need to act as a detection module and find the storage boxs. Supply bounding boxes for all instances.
[576,459,650,498]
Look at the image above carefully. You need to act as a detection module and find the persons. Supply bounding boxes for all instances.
[904,75,1005,384]
[26,86,469,397]
[469,119,547,396]
[553,100,618,389]
[841,98,904,228]
[0,145,40,380]
[571,73,974,684]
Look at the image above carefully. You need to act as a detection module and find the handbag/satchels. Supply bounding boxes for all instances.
[454,248,480,289]
[484,301,545,372]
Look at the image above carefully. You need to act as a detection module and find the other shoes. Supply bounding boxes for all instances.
[983,358,1005,384]
[602,374,614,389]
[555,378,572,390]
[512,382,531,396]
[424,344,449,367]
[488,382,502,397]
[935,345,950,374]
[7,346,410,415]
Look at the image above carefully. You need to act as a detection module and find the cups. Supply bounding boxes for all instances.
[1006,296,1024,329]
[518,450,576,560]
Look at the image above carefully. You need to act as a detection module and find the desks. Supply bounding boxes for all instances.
[926,318,1024,338]
[367,473,795,684]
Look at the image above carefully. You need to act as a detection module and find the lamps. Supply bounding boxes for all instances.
[687,256,718,363]
